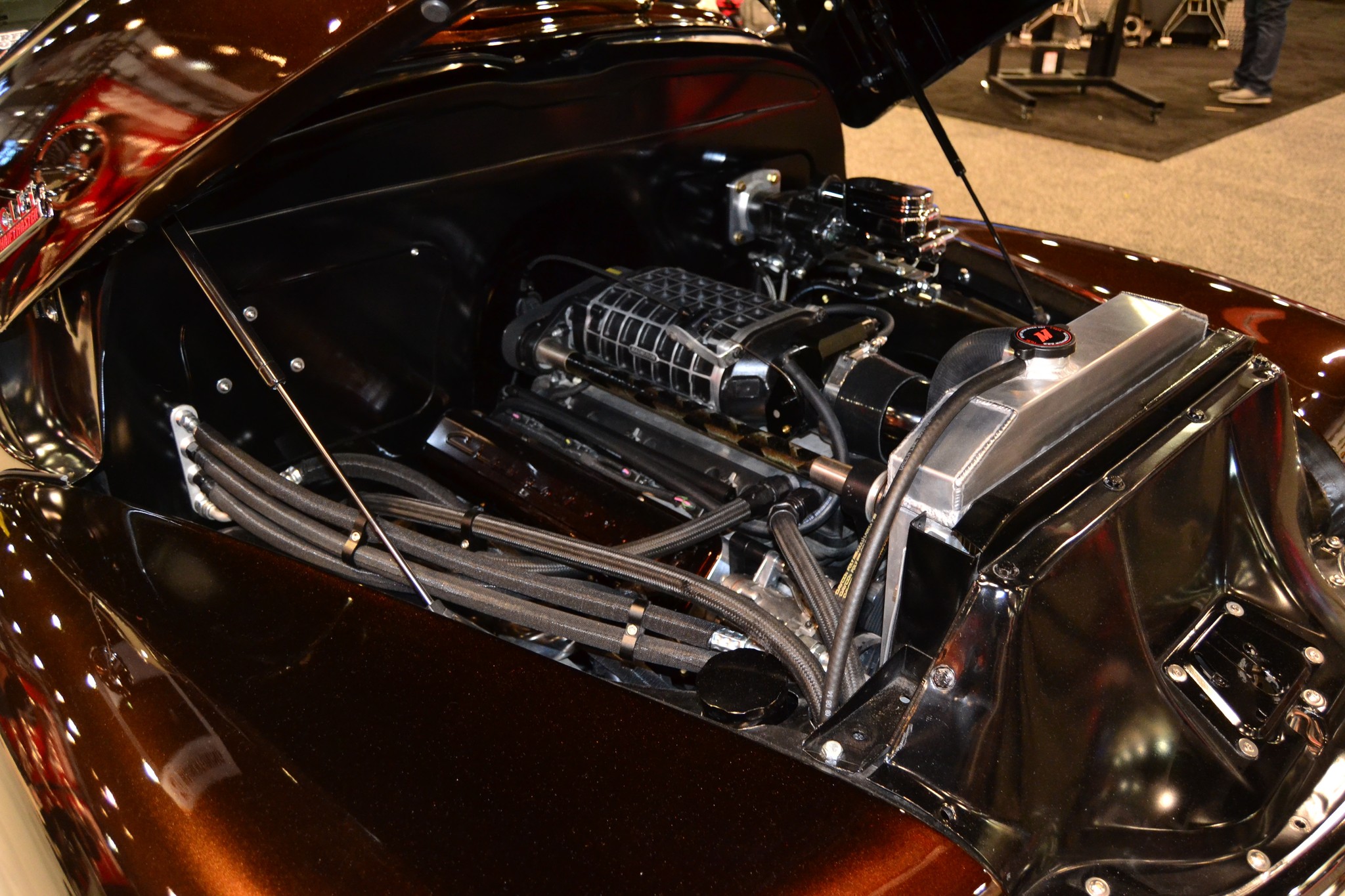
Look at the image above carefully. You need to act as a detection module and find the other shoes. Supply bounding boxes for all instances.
[1217,88,1272,105]
[1208,77,1240,94]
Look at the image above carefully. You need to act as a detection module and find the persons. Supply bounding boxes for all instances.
[1208,0,1293,105]
